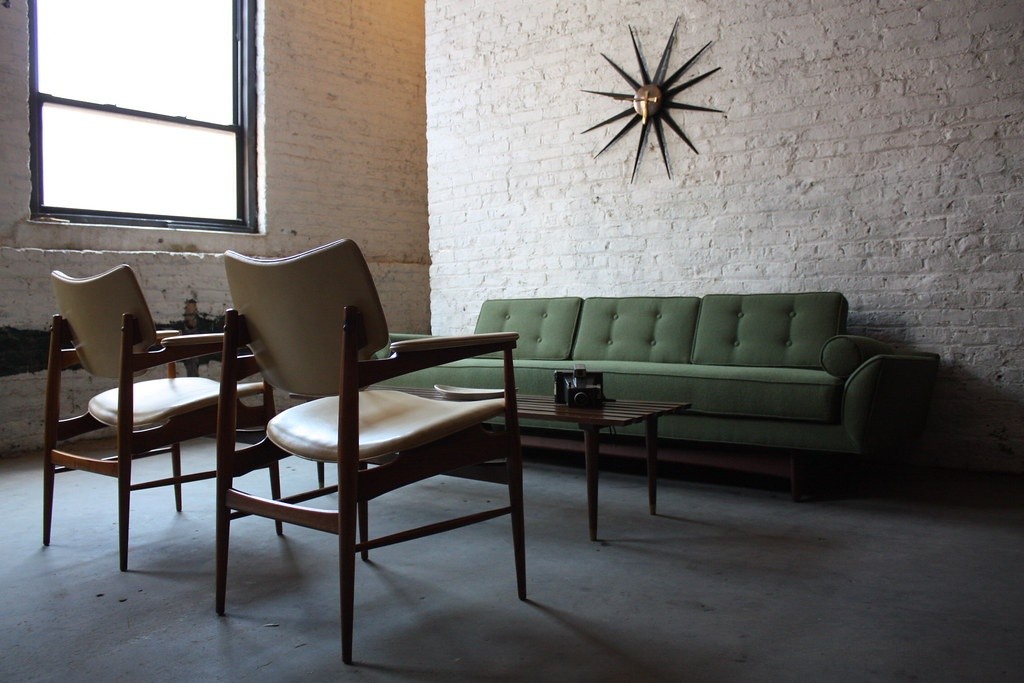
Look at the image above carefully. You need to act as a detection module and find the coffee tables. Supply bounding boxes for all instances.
[289,387,694,542]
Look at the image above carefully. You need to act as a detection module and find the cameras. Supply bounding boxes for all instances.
[552,364,604,409]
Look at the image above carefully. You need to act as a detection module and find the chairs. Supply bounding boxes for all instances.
[41,263,285,572]
[214,237,527,663]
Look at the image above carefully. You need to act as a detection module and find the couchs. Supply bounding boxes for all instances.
[369,292,940,501]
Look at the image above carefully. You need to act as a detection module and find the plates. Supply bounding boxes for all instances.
[434,384,519,400]
